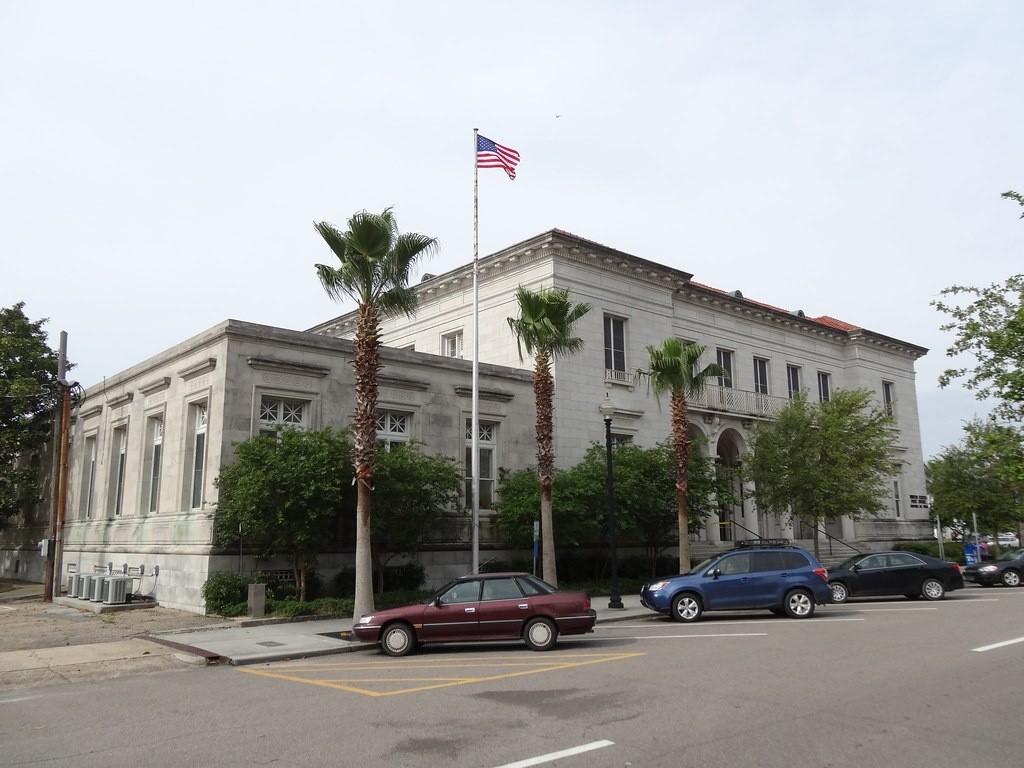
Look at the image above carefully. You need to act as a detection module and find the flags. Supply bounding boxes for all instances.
[475,134,521,181]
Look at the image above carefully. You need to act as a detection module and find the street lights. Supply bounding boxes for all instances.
[598,392,624,608]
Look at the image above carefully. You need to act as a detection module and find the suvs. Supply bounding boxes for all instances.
[639,538,834,622]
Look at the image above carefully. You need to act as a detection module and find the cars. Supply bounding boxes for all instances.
[821,549,966,603]
[352,572,598,657]
[961,546,1024,589]
